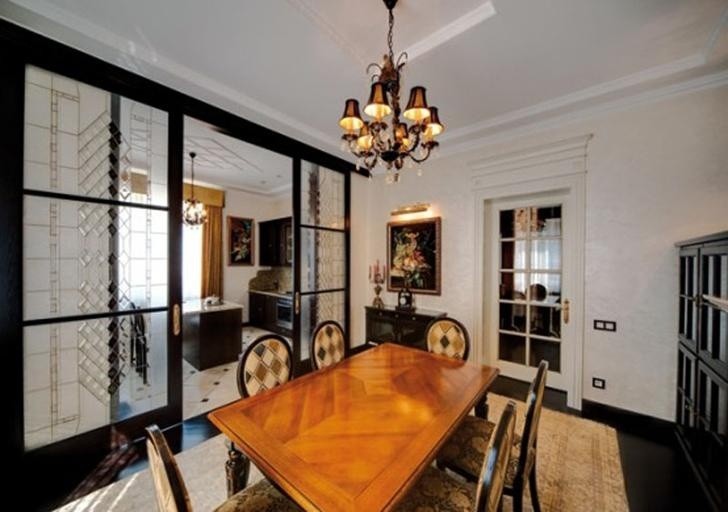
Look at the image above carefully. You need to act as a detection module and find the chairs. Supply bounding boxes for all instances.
[236,334,294,398]
[425,317,471,361]
[435,360,548,512]
[389,401,517,512]
[310,321,347,372]
[144,424,307,512]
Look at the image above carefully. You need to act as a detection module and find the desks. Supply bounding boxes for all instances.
[365,305,448,352]
[183,297,244,371]
[207,342,501,511]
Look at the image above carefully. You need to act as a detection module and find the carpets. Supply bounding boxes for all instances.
[210,391,629,512]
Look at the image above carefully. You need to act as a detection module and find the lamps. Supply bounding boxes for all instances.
[390,204,429,216]
[183,152,207,225]
[339,0,443,183]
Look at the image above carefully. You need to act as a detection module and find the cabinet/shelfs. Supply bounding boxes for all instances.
[675,231,728,511]
[259,217,293,267]
[248,289,293,337]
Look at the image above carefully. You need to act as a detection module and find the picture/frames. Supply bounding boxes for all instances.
[387,216,442,295]
[227,215,255,266]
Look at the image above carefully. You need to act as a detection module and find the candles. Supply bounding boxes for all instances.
[368,260,385,280]
